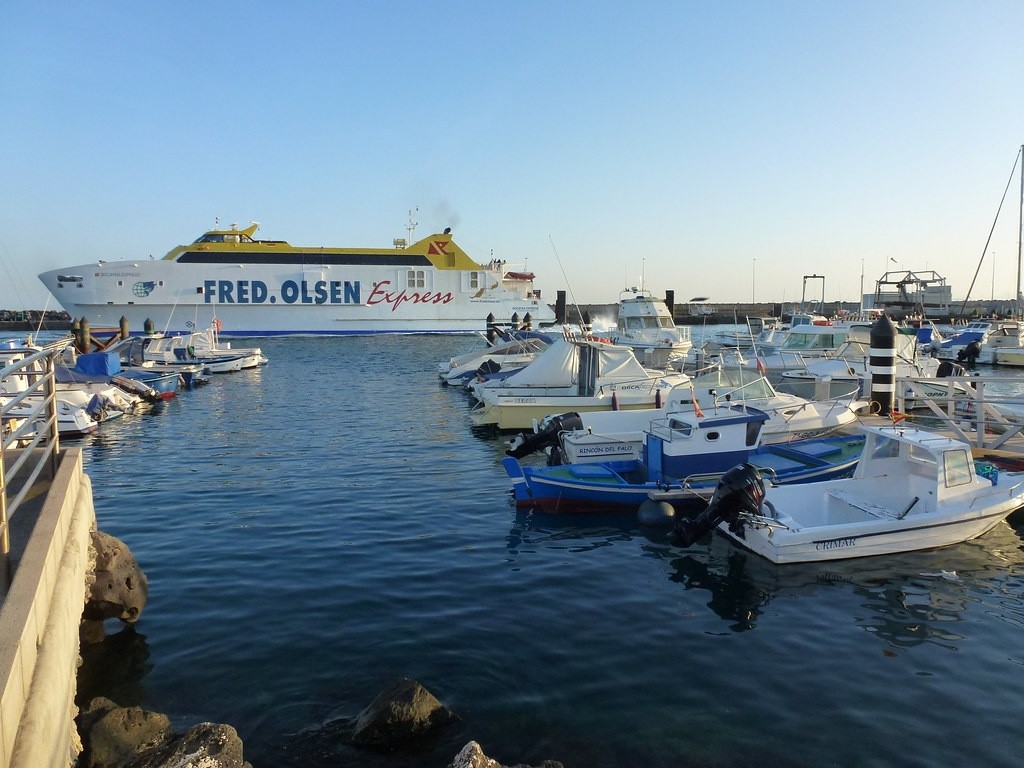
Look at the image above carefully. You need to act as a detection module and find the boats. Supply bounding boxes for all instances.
[182,332,268,367]
[37,216,557,340]
[467,316,692,431]
[700,140,1024,411]
[68,336,182,398]
[499,370,876,460]
[145,339,246,373]
[501,385,894,514]
[0,340,160,438]
[590,289,693,366]
[635,425,1024,565]
[440,328,548,389]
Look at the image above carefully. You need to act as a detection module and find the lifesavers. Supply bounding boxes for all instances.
[217,319,221,332]
[812,319,833,326]
[583,335,612,344]
